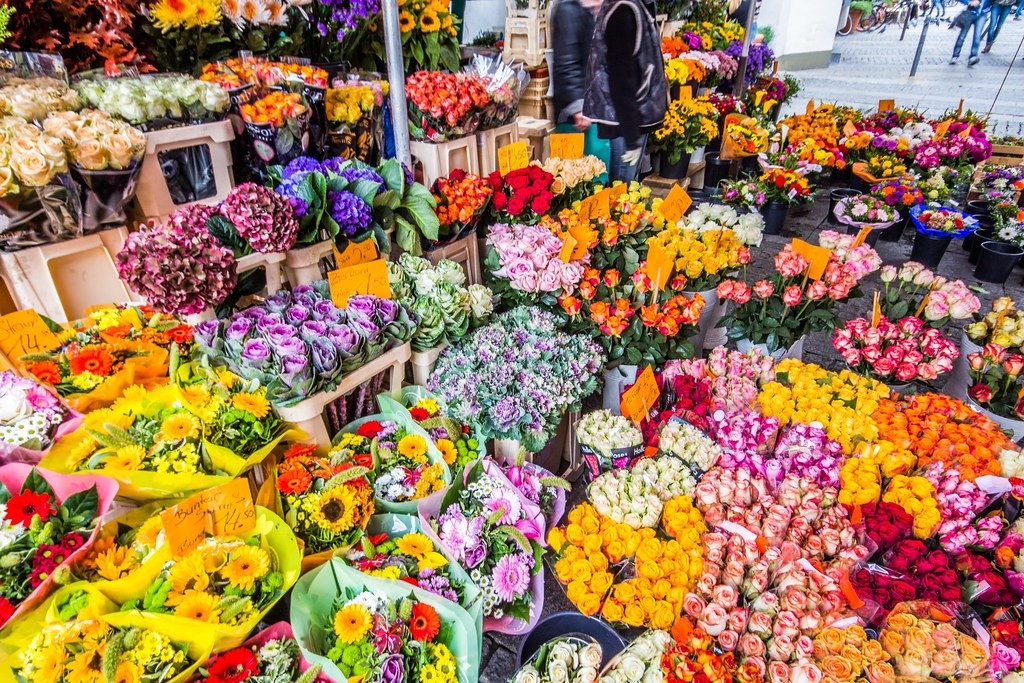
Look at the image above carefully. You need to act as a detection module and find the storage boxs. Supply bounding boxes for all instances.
[541,93,554,119]
[516,95,547,118]
[964,165,1021,204]
[522,85,549,96]
[506,0,549,18]
[424,228,482,287]
[1,223,148,323]
[976,143,1024,165]
[527,66,551,78]
[278,237,334,293]
[409,130,481,191]
[127,117,238,221]
[472,118,519,179]
[516,115,556,167]
[501,16,551,66]
[402,342,451,390]
[273,337,412,460]
[526,76,550,87]
[180,246,287,328]
[484,399,588,480]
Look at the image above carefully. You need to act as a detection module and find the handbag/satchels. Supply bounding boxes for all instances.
[948,4,974,30]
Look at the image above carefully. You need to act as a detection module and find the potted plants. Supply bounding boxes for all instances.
[841,0,873,34]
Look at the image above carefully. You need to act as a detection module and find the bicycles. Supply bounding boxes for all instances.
[836,0,958,36]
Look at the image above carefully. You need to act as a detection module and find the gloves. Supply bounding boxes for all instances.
[621,147,642,166]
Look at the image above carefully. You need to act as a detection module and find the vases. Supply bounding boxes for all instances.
[910,231,952,272]
[315,60,347,87]
[965,386,1024,445]
[517,611,627,677]
[802,172,817,191]
[736,330,806,365]
[736,155,765,181]
[601,364,638,414]
[765,103,782,122]
[663,19,685,37]
[790,194,812,217]
[668,78,700,101]
[544,47,554,95]
[846,225,880,248]
[677,285,719,358]
[969,227,997,264]
[704,150,733,188]
[759,201,789,235]
[683,190,708,214]
[879,211,910,243]
[973,241,1023,283]
[851,175,871,194]
[697,85,719,96]
[939,331,1012,410]
[712,195,748,221]
[817,164,838,188]
[688,144,706,164]
[965,199,989,216]
[961,212,995,252]
[658,147,693,179]
[701,268,742,350]
[828,187,863,227]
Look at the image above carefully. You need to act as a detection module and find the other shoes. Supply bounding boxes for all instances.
[968,57,980,65]
[919,12,925,17]
[1013,17,1021,21]
[981,41,992,53]
[950,57,958,64]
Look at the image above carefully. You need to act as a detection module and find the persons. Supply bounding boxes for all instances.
[900,0,1016,65]
[582,1,670,188]
[551,1,611,186]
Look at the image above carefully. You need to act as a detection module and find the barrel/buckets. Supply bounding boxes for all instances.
[973,241,1024,285]
[827,189,862,227]
[684,191,711,217]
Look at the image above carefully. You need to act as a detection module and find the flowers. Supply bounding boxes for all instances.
[0,0,1024,683]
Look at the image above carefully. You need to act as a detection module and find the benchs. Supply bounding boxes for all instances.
[640,161,706,211]
